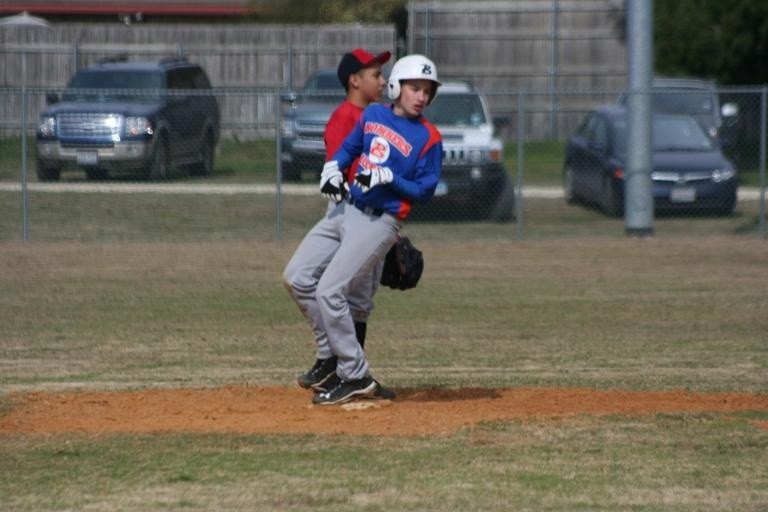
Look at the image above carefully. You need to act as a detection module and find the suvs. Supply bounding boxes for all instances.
[616,77,739,152]
[418,77,513,225]
[33,55,223,181]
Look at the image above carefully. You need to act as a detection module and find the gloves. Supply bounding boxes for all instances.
[352,165,394,194]
[319,159,351,204]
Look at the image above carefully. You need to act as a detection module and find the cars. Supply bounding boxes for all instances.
[279,68,392,181]
[564,104,736,218]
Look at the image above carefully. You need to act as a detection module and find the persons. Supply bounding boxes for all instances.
[320,48,398,400]
[281,53,442,405]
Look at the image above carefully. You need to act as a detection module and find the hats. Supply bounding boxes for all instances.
[336,47,391,85]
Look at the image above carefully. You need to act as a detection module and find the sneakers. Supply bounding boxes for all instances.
[296,355,338,391]
[357,376,397,401]
[311,369,377,407]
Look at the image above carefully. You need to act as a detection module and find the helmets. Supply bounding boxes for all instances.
[386,54,442,108]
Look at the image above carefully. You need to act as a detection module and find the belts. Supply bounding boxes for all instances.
[343,191,384,217]
